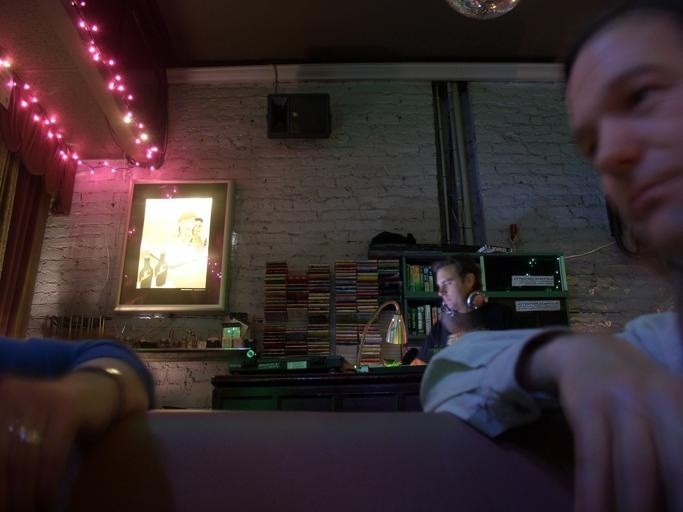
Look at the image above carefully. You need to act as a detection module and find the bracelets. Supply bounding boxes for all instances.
[76,364,126,433]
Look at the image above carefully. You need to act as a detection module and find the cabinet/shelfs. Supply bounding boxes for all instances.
[212,373,424,411]
[402,252,571,340]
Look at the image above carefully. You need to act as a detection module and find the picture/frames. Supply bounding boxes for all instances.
[113,179,236,314]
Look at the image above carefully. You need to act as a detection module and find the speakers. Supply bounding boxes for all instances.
[267,94,331,139]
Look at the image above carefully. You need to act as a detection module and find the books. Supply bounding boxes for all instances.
[258,256,441,362]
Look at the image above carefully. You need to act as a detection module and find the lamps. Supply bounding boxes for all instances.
[353,300,407,374]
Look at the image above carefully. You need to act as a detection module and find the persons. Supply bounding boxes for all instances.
[419,4,683,511]
[407,256,536,365]
[1,334,156,511]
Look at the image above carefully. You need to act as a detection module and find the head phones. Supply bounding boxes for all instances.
[441,290,489,316]
